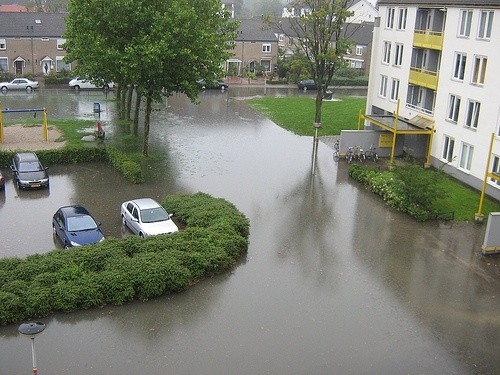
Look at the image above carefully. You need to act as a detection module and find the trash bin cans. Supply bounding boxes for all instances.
[93,102,100,113]
[324,90,333,100]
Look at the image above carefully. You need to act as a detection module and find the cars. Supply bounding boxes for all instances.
[52,205,106,251]
[0,78,39,91]
[196,78,229,90]
[297,80,325,90]
[121,198,179,238]
[69,74,115,89]
[10,152,49,190]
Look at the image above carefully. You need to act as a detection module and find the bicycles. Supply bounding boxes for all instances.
[365,144,379,164]
[344,145,355,167]
[334,140,339,151]
[352,145,365,165]
